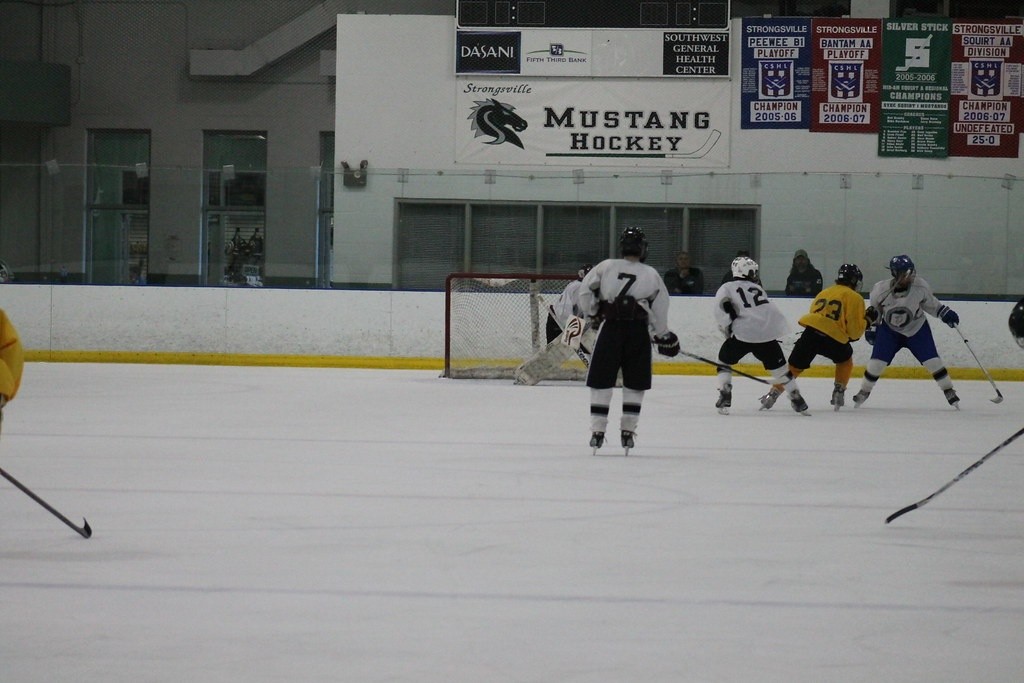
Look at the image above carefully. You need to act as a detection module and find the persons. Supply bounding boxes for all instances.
[758,264,878,413]
[853,255,961,412]
[714,257,811,417]
[664,252,703,296]
[579,227,681,456]
[546,264,592,344]
[0,308,24,430]
[783,249,823,297]
[721,250,762,288]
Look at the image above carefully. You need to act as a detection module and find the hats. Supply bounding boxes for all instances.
[791,249,808,263]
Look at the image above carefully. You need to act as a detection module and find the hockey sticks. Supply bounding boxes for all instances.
[536,294,623,389]
[678,349,793,385]
[883,427,1024,525]
[952,322,1004,404]
[877,269,910,306]
[0,466,93,540]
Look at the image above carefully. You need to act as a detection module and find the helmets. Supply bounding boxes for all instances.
[1007,300,1024,349]
[619,225,650,262]
[731,255,760,280]
[577,263,593,281]
[835,263,864,293]
[890,254,916,284]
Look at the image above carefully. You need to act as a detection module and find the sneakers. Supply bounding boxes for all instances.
[621,430,638,456]
[715,383,733,414]
[789,391,811,417]
[851,389,871,408]
[758,385,785,411]
[589,431,608,457]
[830,382,847,411]
[944,388,961,411]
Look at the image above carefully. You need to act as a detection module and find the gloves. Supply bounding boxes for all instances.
[654,332,682,360]
[865,326,876,345]
[589,309,603,332]
[863,306,878,330]
[936,305,960,329]
